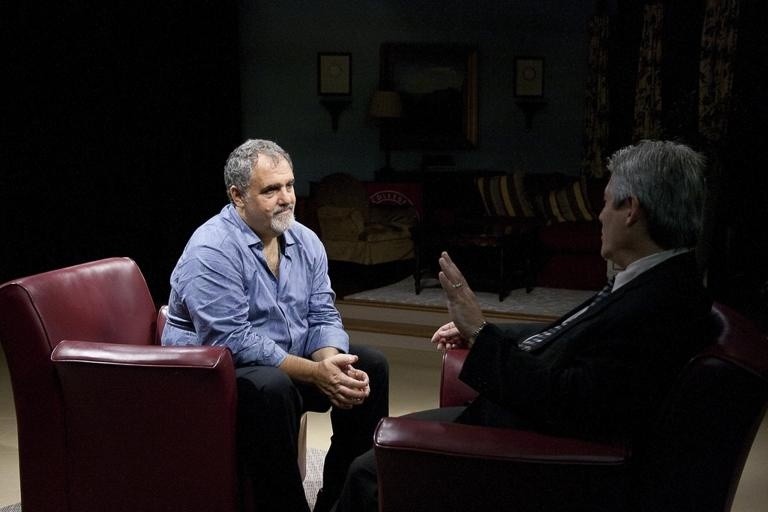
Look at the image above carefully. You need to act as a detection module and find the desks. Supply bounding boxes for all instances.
[414,218,547,303]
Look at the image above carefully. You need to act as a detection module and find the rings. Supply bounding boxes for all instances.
[453,281,461,288]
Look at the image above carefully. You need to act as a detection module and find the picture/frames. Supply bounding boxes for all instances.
[317,51,353,97]
[513,56,544,98]
[378,42,478,152]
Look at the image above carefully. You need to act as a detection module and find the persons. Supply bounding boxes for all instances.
[159,135,393,512]
[332,134,726,512]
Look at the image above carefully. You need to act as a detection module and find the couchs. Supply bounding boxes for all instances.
[435,173,608,290]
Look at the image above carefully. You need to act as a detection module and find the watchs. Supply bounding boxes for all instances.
[471,321,487,337]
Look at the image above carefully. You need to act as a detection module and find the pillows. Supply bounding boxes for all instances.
[472,174,540,219]
[537,175,596,227]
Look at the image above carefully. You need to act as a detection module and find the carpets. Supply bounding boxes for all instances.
[343,274,598,320]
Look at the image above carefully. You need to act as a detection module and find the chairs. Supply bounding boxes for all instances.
[0,256,307,510]
[373,301,768,512]
[305,173,417,290]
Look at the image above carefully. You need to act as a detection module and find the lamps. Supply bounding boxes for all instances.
[368,91,402,173]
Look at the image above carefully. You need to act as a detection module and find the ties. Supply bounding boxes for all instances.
[517,276,615,352]
